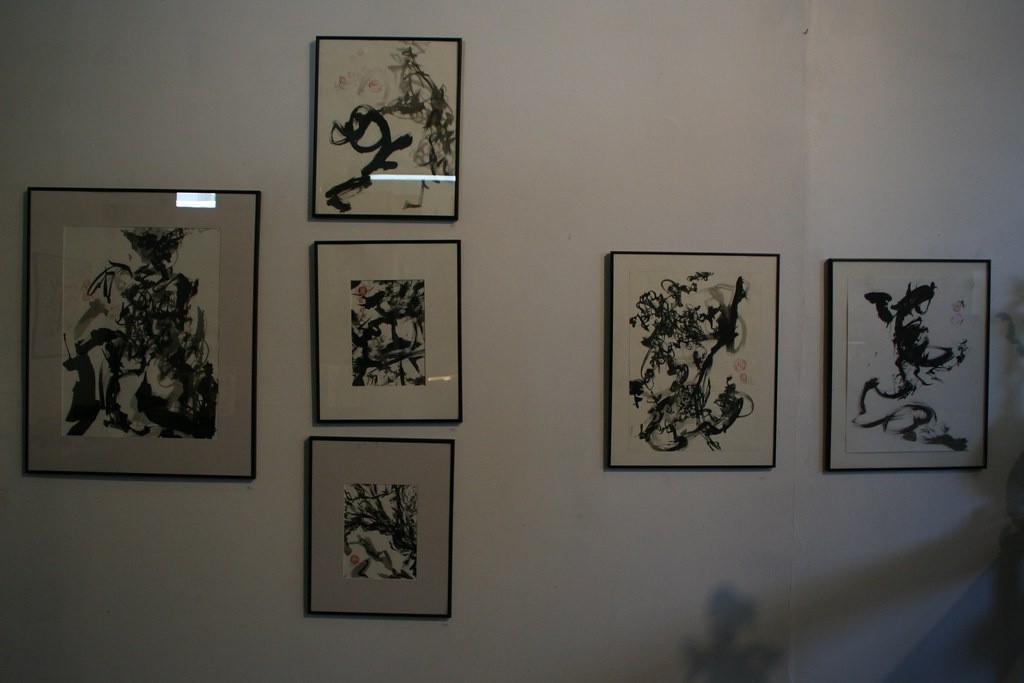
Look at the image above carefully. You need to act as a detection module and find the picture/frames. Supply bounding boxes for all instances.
[823,258,992,472]
[308,435,454,620]
[22,186,261,478]
[313,240,464,426]
[312,34,462,222]
[607,251,780,470]
